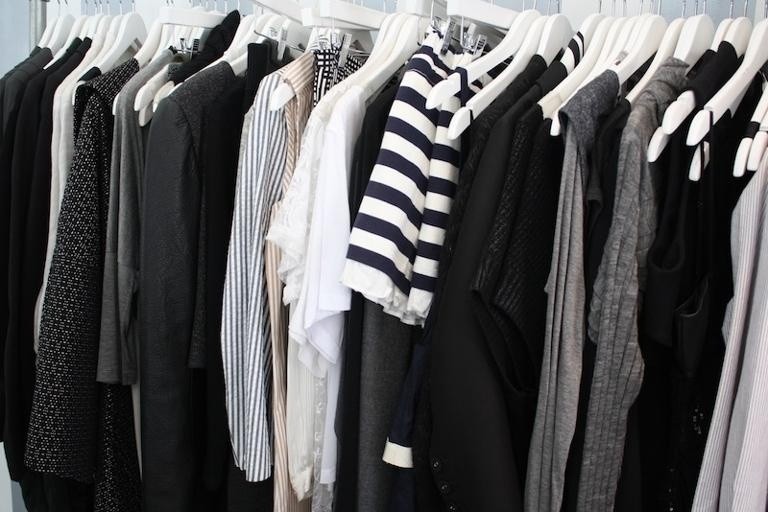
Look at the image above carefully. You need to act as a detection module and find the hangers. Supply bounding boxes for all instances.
[34,0,768,184]
